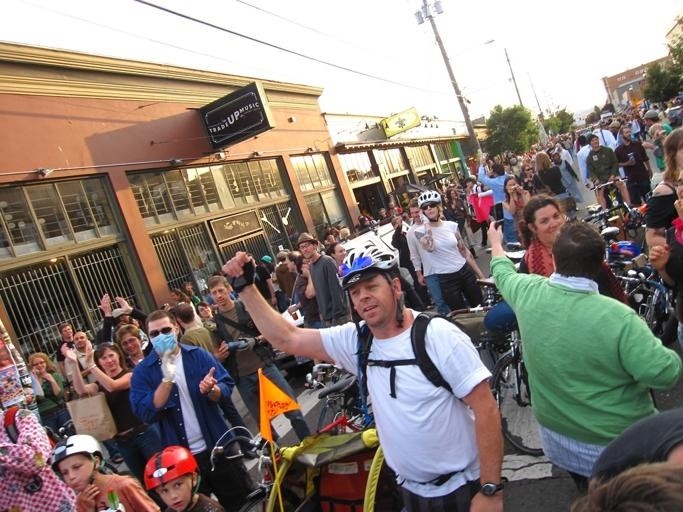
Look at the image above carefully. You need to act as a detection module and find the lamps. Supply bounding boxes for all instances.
[38,168,54,177]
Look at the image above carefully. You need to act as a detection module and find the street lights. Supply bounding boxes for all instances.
[414,0,481,156]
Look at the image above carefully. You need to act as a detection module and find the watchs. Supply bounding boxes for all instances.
[479,480,504,495]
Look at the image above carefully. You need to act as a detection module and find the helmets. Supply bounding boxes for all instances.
[417,190,442,210]
[143,446,199,491]
[48,435,109,481]
[336,237,401,294]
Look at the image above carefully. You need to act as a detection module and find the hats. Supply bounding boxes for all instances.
[642,110,658,119]
[608,121,621,129]
[295,233,319,247]
[112,308,125,319]
[259,255,272,263]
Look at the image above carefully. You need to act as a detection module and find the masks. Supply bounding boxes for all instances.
[149,333,179,354]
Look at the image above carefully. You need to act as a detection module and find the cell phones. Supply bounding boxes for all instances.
[494,201,505,234]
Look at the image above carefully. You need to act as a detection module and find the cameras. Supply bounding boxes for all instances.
[628,152,634,161]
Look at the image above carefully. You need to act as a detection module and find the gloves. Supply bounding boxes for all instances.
[160,350,177,382]
[238,337,257,353]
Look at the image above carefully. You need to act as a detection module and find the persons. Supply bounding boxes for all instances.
[518,195,626,304]
[223,240,506,510]
[573,407,683,512]
[594,96,682,320]
[1,276,310,512]
[467,132,615,253]
[487,217,682,490]
[254,226,352,384]
[358,178,492,314]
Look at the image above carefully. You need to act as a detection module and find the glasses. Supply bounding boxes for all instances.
[422,202,436,211]
[336,256,382,278]
[149,326,174,336]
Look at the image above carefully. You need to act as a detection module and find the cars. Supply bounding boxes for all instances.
[343,219,411,292]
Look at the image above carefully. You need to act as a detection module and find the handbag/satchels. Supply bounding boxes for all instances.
[281,303,304,327]
[552,192,576,213]
[65,391,119,442]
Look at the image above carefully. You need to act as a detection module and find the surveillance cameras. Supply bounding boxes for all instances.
[464,97,471,103]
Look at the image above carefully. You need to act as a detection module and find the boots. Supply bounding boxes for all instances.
[470,248,477,258]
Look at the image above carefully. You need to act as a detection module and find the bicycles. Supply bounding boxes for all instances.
[209,363,377,512]
